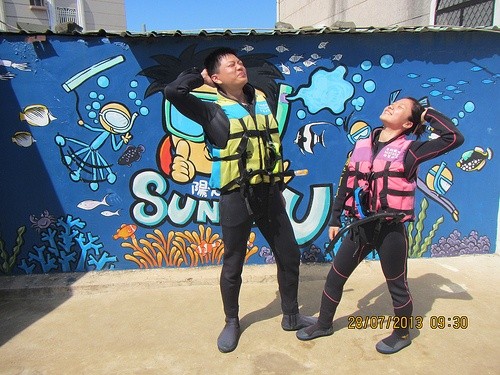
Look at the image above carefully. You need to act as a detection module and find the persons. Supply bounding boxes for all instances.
[164,47,317,353]
[296,95,464,355]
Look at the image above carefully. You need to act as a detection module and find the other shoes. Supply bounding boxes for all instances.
[281,314,319,331]
[296,322,334,341]
[376,330,411,354]
[216,317,241,353]
